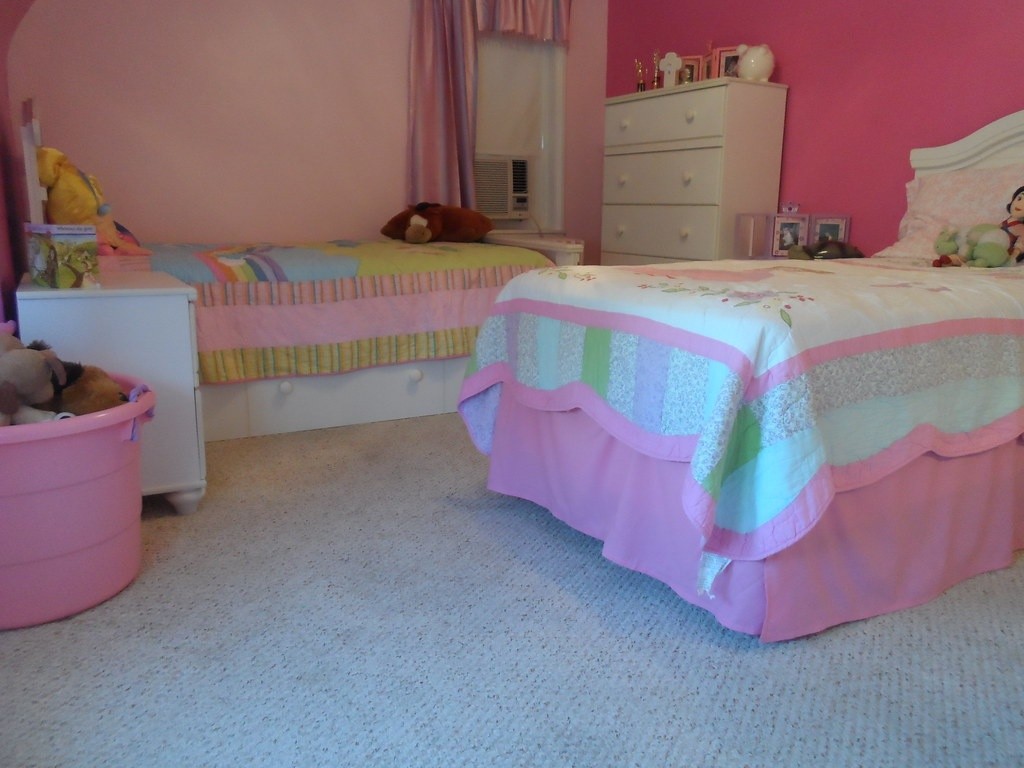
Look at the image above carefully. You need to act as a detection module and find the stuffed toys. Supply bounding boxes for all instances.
[36,144,152,257]
[930,224,1011,268]
[0,320,131,428]
[931,186,1024,267]
[379,201,496,244]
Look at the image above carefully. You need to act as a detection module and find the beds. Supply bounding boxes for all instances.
[48,230,557,444]
[455,243,1024,644]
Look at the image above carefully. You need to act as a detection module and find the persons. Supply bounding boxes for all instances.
[781,227,793,248]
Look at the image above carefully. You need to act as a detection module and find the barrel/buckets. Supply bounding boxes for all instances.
[0,354,163,629]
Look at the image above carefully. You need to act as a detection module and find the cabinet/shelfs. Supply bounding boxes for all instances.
[599,76,790,265]
[15,269,208,515]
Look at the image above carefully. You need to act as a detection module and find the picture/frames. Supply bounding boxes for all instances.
[809,213,851,253]
[772,214,808,256]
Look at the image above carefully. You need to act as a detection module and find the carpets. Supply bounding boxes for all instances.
[2,415,1024,768]
